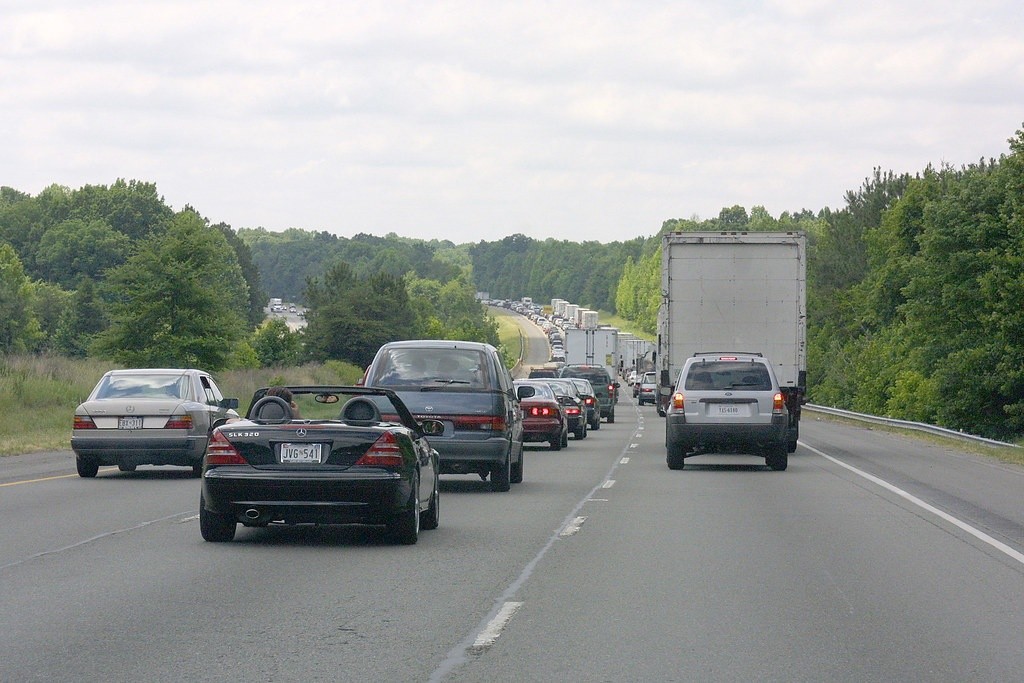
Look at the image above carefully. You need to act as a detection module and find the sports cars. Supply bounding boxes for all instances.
[200,385,444,546]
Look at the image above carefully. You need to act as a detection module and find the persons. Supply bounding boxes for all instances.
[264,386,303,420]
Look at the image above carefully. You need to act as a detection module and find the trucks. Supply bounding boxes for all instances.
[652,230,808,454]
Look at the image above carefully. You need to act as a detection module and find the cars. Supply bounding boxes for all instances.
[563,378,603,430]
[512,381,571,451]
[637,371,656,406]
[474,290,654,404]
[527,377,590,440]
[70,368,240,479]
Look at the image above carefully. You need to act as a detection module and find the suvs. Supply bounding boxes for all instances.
[559,363,620,424]
[363,340,535,493]
[660,351,790,471]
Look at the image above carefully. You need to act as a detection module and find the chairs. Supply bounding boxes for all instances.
[336,396,383,421]
[249,396,292,420]
[692,372,714,389]
